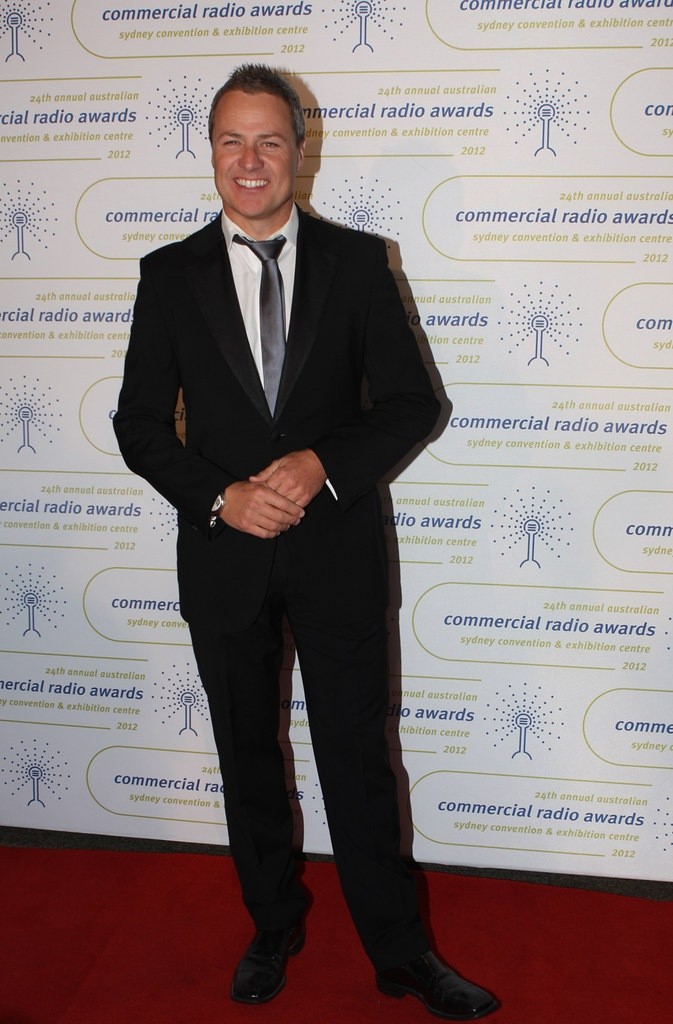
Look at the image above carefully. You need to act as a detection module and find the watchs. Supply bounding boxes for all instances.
[211,488,226,530]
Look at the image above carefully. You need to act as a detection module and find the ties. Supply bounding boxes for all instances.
[232,234,288,419]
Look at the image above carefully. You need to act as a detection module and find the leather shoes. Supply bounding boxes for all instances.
[231,919,307,1005]
[375,950,500,1020]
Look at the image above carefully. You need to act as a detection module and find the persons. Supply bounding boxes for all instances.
[115,64,502,1024]
